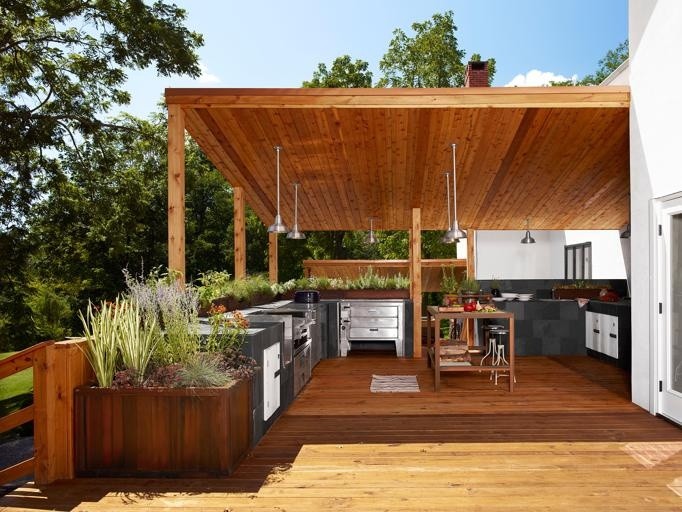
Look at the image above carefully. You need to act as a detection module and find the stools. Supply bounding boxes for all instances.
[479,324,517,386]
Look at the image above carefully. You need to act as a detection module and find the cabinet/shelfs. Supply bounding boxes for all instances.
[341,302,413,357]
[584,304,630,370]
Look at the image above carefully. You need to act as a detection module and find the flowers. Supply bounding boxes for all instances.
[66,255,265,405]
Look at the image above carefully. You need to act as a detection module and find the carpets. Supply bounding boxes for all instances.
[369,373,421,392]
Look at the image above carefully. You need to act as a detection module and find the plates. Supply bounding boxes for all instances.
[492,292,534,302]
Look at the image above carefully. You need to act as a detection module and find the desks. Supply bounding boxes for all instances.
[426,304,515,392]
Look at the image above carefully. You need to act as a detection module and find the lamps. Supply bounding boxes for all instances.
[521,218,535,244]
[441,144,468,244]
[266,144,307,241]
